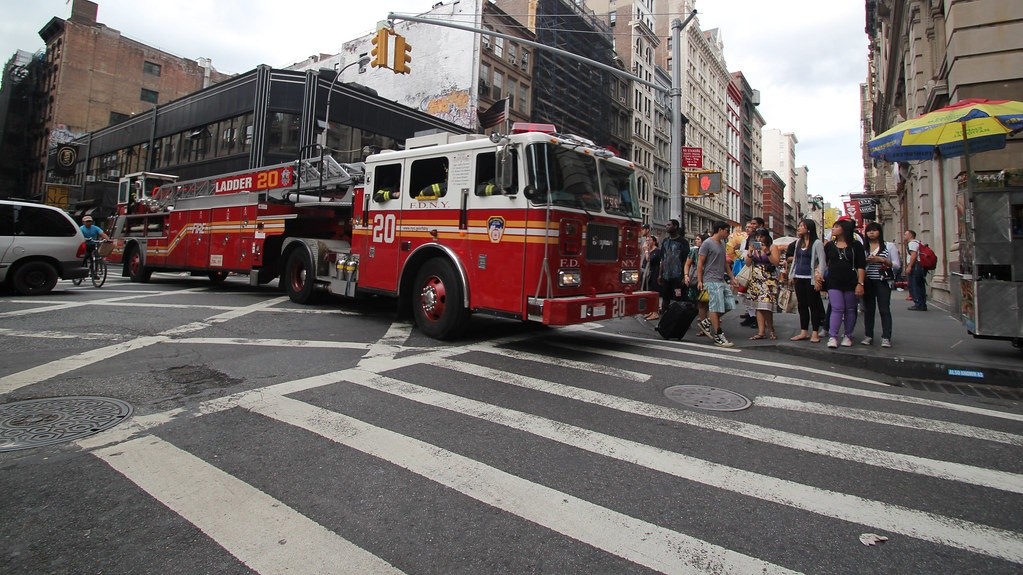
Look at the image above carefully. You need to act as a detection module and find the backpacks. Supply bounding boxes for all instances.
[913,241,937,269]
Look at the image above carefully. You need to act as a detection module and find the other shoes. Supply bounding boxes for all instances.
[908,305,927,311]
[861,336,873,345]
[645,312,659,320]
[818,329,828,337]
[881,339,891,347]
[740,312,758,329]
[841,335,852,346]
[827,337,837,347]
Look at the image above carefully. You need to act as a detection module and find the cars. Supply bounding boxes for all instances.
[0,200,91,295]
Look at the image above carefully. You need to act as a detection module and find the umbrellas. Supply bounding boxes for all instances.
[867,98,1023,202]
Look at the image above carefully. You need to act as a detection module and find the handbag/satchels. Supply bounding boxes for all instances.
[777,285,798,314]
[879,269,895,281]
[842,269,857,290]
[735,265,753,287]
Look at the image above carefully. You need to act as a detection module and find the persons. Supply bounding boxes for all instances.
[80,216,111,279]
[657,219,689,313]
[697,221,738,348]
[130,182,136,210]
[788,220,825,343]
[815,220,864,347]
[475,176,515,196]
[904,230,927,311]
[861,222,901,348]
[374,184,400,202]
[746,228,779,340]
[419,181,448,197]
[640,217,871,336]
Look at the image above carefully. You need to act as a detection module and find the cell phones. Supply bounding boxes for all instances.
[754,242,763,248]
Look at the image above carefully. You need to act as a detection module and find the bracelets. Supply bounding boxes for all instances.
[815,273,818,277]
[395,192,397,199]
[747,253,752,258]
[858,281,864,286]
[766,252,771,256]
[684,274,688,276]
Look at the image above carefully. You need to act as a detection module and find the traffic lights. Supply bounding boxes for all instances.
[395,36,411,76]
[699,172,722,194]
[687,177,700,195]
[371,30,387,68]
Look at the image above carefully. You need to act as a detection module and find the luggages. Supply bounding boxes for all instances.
[655,288,706,340]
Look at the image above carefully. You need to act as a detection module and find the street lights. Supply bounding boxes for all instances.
[323,57,371,150]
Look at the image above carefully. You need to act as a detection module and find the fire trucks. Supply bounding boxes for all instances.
[112,123,661,348]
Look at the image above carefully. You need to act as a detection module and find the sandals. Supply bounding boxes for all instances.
[769,329,777,340]
[749,334,767,340]
[790,334,810,341]
[811,336,820,343]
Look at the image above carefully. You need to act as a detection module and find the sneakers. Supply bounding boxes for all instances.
[698,318,712,335]
[713,332,734,347]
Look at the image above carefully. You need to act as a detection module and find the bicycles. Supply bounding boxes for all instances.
[72,236,114,289]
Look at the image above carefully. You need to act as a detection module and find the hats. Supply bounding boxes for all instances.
[642,224,650,228]
[82,216,93,221]
